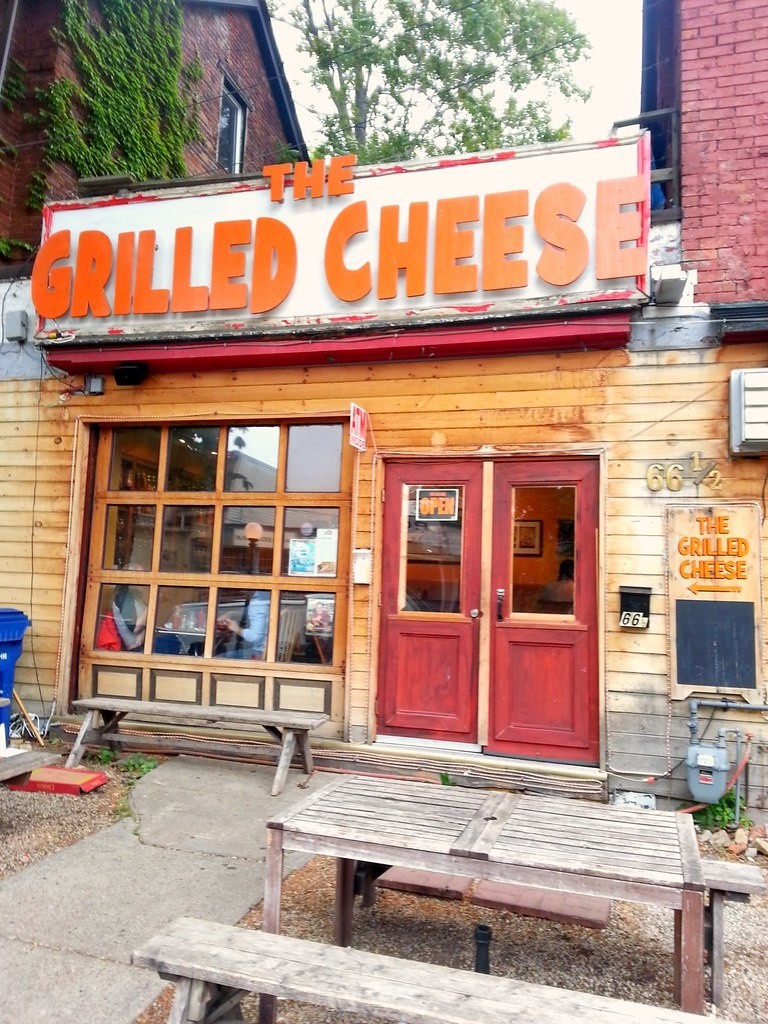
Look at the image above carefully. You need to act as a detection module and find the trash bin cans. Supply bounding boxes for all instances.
[0,608,32,744]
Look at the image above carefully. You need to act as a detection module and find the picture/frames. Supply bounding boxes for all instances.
[513,519,544,558]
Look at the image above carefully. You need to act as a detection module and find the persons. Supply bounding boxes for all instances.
[312,603,329,633]
[113,564,148,653]
[535,560,574,615]
[216,572,270,660]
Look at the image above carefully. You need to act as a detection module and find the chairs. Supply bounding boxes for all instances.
[260,604,303,663]
[98,609,127,651]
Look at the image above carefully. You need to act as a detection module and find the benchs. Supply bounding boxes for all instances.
[701,857,768,1006]
[63,695,331,793]
[132,918,753,1024]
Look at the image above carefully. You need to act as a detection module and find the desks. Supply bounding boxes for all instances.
[257,774,707,1024]
[155,625,227,657]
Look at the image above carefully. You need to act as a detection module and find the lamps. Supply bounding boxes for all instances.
[112,365,144,385]
